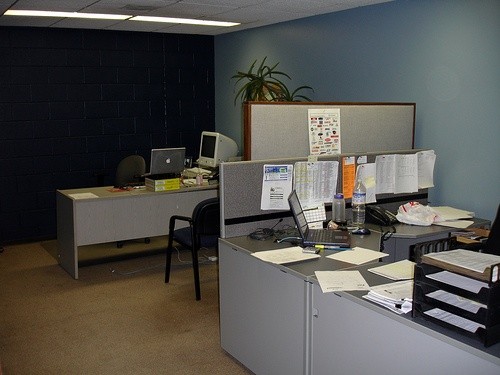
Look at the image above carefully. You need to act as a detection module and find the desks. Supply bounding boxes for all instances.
[219,217,500,375]
[56,179,219,281]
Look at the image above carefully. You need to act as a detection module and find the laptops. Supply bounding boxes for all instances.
[141,147,186,177]
[288,190,351,248]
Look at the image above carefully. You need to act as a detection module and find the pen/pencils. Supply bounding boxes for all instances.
[313,245,352,250]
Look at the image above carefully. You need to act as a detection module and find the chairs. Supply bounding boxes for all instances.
[94,155,151,249]
[164,197,220,301]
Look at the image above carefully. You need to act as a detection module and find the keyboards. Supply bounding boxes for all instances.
[187,167,211,174]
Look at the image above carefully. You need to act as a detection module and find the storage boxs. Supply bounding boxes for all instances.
[145,177,180,193]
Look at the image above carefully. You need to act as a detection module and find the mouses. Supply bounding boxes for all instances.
[352,227,371,235]
[201,173,209,176]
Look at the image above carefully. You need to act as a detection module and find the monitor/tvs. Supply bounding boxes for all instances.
[198,131,238,167]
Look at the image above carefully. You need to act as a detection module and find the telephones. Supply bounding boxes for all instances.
[365,205,400,226]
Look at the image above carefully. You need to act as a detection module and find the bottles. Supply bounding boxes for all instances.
[333,195,345,223]
[196,169,204,186]
[351,179,367,227]
